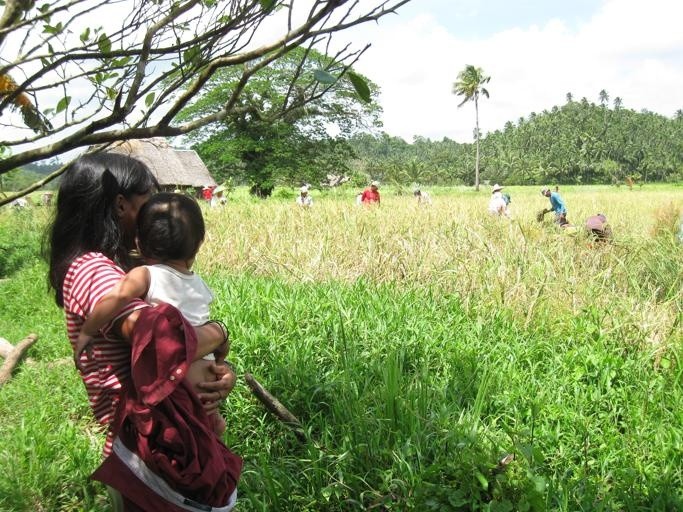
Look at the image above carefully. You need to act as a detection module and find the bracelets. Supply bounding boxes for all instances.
[211,318,229,342]
[204,320,225,346]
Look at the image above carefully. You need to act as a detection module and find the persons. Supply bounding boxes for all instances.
[73,193,225,441]
[585,213,613,243]
[40,153,240,511]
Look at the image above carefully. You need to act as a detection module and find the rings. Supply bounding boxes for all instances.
[218,392,224,399]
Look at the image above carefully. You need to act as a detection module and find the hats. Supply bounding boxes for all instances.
[300,186,309,193]
[540,185,550,197]
[492,183,505,193]
[372,181,381,189]
